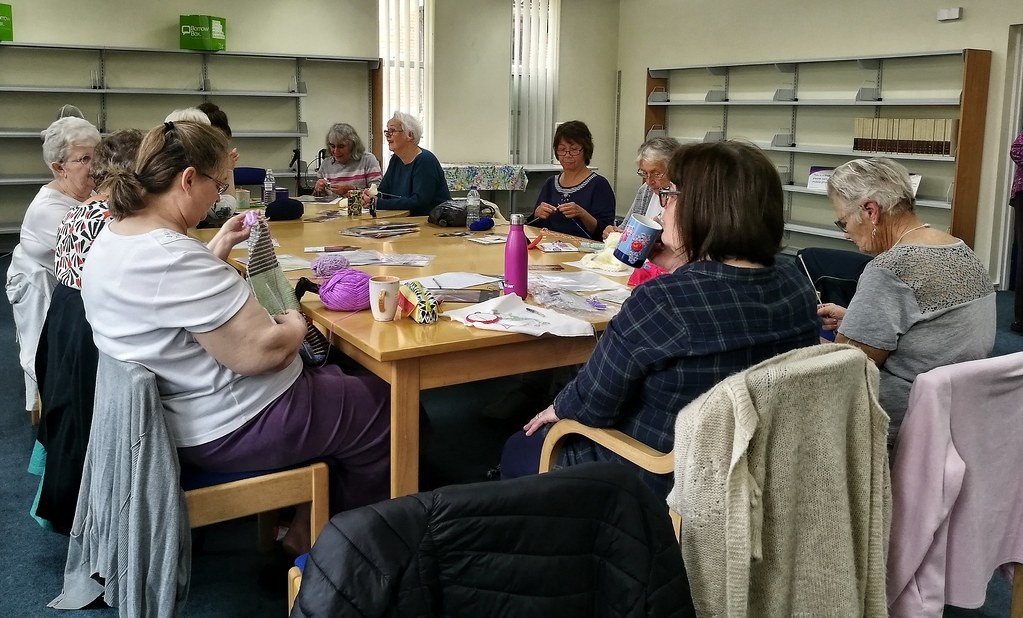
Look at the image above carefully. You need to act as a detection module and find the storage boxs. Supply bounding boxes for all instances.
[0,3,16,44]
[177,13,232,52]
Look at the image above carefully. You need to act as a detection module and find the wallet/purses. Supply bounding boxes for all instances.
[401,280,438,325]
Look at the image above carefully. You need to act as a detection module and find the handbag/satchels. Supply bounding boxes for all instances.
[179,15,226,50]
[428,199,495,227]
[0,3,13,41]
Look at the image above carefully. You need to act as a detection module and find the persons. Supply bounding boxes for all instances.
[1008,131,1023,334]
[532,120,616,242]
[18,102,239,288]
[499,143,819,513]
[313,123,383,197]
[81,121,428,573]
[603,137,682,240]
[817,160,997,455]
[360,111,451,217]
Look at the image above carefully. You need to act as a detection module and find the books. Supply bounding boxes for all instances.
[853,117,959,158]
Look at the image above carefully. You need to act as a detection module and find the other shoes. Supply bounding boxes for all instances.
[1010,321,1023,333]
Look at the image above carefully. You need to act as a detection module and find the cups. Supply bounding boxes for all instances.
[275,187,289,202]
[612,213,663,269]
[261,186,265,201]
[236,190,250,209]
[369,276,401,322]
[348,189,363,216]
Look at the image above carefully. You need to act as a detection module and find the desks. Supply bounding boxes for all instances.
[180,177,654,503]
[432,152,607,217]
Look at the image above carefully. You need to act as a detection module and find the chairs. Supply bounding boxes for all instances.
[278,464,661,617]
[536,341,897,618]
[92,351,331,580]
[794,237,885,328]
[889,349,1023,617]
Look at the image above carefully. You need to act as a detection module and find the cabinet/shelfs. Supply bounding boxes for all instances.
[637,44,992,270]
[0,36,387,269]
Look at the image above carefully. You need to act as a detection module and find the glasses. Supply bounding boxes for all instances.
[59,155,92,167]
[833,214,850,235]
[555,146,584,158]
[200,170,229,195]
[384,128,403,137]
[659,186,681,208]
[637,163,668,181]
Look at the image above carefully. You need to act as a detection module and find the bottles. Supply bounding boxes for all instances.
[264,169,276,207]
[466,185,480,230]
[504,214,528,301]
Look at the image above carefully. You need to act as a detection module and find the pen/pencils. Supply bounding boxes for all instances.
[432,277,443,289]
[526,306,545,316]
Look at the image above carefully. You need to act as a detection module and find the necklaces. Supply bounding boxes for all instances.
[892,223,931,246]
